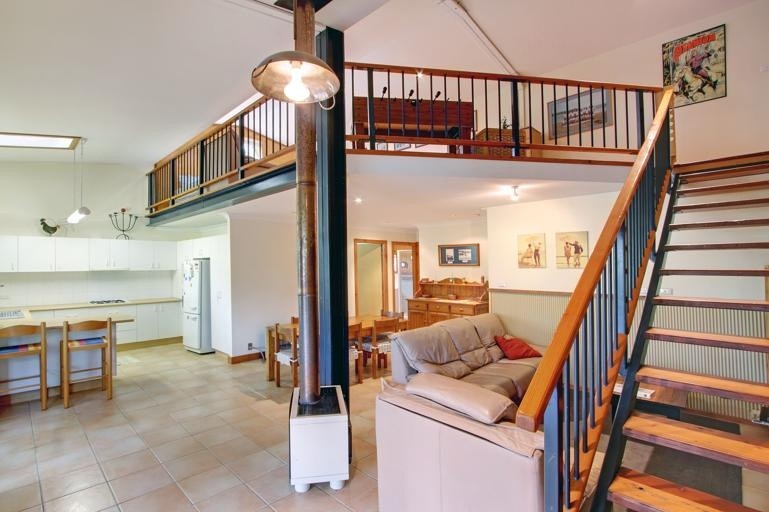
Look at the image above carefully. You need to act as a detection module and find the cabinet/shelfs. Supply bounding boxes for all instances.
[521,125,543,159]
[137,301,182,342]
[0,234,177,272]
[29,310,54,323]
[405,278,489,331]
[54,308,101,323]
[101,304,137,344]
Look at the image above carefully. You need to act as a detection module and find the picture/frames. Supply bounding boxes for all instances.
[547,88,614,140]
[373,141,427,150]
[437,243,480,267]
[472,108,479,132]
[661,23,727,110]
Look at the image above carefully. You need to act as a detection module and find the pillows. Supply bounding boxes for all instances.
[494,336,543,361]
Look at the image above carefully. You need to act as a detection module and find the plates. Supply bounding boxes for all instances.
[422,295,432,297]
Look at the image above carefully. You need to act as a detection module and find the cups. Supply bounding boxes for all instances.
[448,294,464,300]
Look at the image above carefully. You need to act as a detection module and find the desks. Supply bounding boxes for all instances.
[1,316,135,407]
[607,377,692,429]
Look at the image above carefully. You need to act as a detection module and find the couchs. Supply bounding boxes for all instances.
[386,311,562,401]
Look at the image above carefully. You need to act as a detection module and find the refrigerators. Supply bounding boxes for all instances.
[181,257,215,355]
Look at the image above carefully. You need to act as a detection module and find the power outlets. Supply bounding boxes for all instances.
[248,343,252,350]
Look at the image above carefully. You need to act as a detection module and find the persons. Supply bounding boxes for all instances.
[518,241,543,266]
[563,240,584,268]
[685,45,712,82]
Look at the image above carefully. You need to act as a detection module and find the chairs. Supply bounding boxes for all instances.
[266,311,409,390]
[373,373,580,511]
[58,318,114,408]
[0,323,49,412]
[200,123,278,186]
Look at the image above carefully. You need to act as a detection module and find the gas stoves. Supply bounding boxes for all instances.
[89,299,126,305]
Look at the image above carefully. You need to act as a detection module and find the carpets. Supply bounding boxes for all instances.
[641,411,745,509]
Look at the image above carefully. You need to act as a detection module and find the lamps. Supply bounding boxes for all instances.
[64,138,92,226]
[249,0,342,107]
